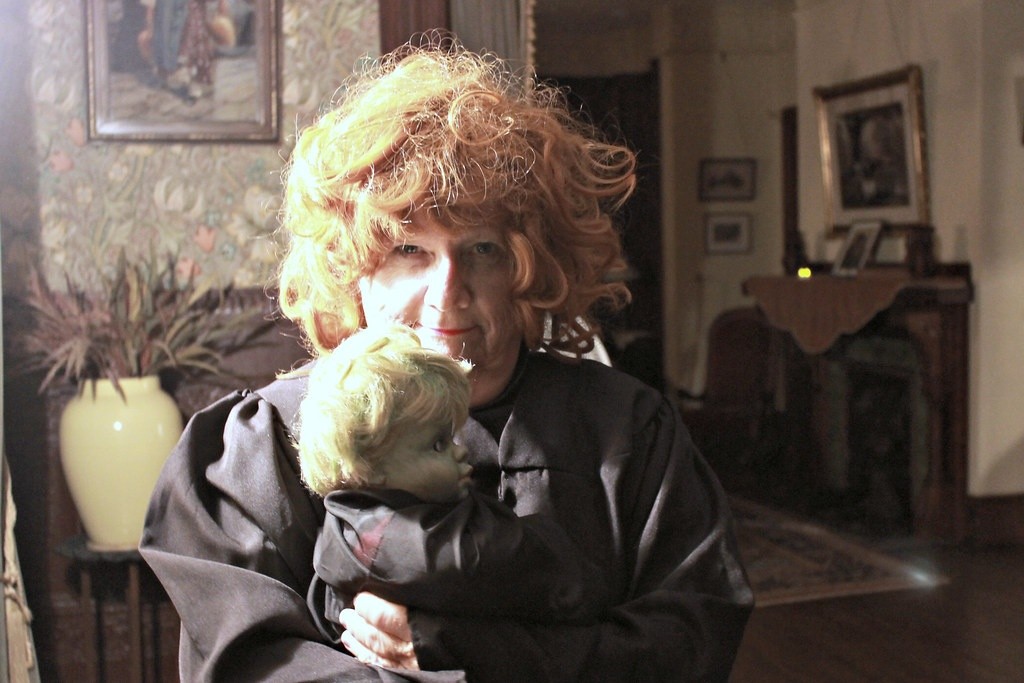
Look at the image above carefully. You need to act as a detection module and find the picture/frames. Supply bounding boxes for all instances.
[831,219,888,278]
[697,157,757,204]
[82,0,281,141]
[812,63,931,241]
[704,211,752,255]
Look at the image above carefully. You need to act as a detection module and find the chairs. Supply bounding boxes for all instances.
[677,305,768,489]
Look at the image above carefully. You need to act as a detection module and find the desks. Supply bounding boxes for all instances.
[62,534,156,683]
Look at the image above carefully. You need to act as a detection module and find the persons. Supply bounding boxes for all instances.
[301,320,601,657]
[140,52,754,683]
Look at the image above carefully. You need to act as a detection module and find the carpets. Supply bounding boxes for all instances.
[726,495,949,609]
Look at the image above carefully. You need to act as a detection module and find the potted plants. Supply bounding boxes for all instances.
[3,240,281,552]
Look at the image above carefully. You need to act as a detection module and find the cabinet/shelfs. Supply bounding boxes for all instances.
[753,277,975,543]
